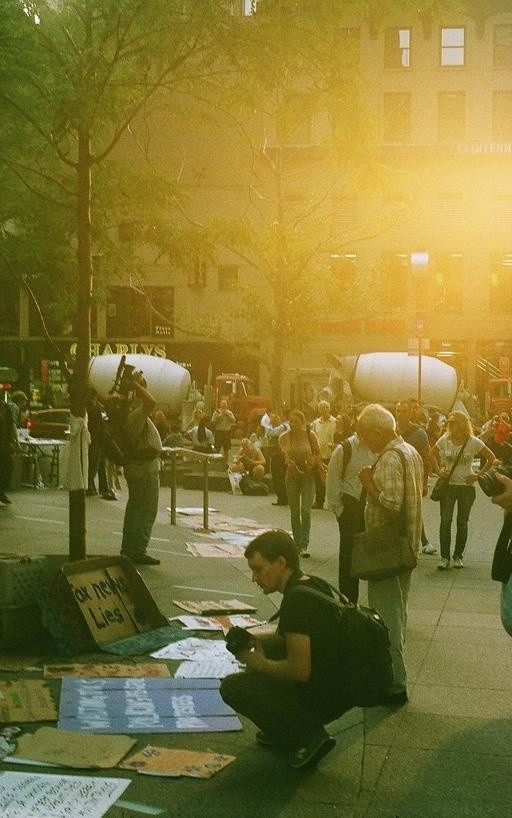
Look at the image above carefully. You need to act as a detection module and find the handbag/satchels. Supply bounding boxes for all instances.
[430,478,449,501]
[238,477,269,495]
[315,461,328,487]
[350,520,418,581]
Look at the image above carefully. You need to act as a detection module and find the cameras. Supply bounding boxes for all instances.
[223,624,253,655]
[478,464,510,496]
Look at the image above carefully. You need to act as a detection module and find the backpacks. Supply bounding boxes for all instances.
[88,419,127,465]
[286,573,394,708]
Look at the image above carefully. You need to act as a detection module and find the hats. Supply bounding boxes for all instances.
[14,391,29,401]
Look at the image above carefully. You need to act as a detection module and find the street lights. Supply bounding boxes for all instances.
[408,230,432,404]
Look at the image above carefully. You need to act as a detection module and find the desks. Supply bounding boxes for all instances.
[18,438,66,486]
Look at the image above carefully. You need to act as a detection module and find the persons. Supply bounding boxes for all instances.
[89,373,512,710]
[0,399,12,504]
[218,529,352,771]
[7,391,28,451]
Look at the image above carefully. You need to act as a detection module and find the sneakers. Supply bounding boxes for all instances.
[422,543,437,554]
[287,729,336,769]
[255,728,280,746]
[298,548,310,558]
[454,558,464,568]
[382,693,405,705]
[437,557,450,569]
[126,551,160,565]
